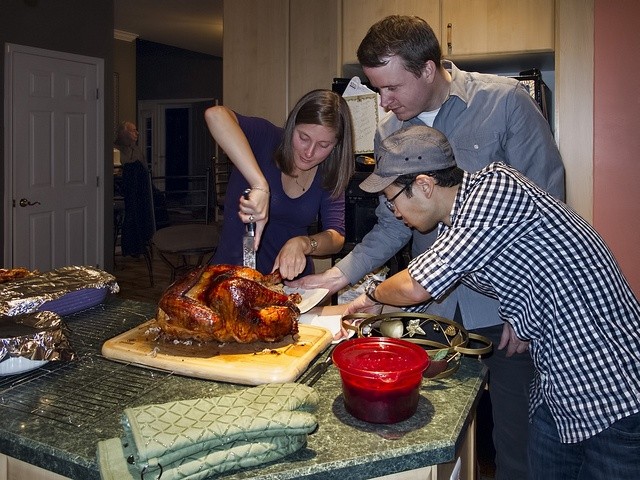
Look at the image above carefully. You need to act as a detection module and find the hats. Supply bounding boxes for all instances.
[359,124,456,193]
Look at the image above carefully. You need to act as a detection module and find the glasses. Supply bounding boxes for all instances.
[385,173,433,211]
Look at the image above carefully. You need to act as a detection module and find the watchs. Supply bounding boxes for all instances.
[306,234,318,256]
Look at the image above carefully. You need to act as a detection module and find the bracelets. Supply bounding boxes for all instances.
[250,186,270,195]
[364,278,383,305]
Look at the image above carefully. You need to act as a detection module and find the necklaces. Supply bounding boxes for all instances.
[294,168,312,192]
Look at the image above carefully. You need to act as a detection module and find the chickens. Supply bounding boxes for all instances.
[155,248,302,345]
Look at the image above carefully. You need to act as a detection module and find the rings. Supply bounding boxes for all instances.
[249,214,255,223]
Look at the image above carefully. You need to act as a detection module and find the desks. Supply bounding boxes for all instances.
[147,222,221,281]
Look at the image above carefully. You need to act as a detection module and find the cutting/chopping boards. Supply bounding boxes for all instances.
[101,318,333,385]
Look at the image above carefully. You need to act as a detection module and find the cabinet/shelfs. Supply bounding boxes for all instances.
[341,0,555,65]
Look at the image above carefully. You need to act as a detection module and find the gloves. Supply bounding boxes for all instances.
[97,434,306,480]
[121,383,319,480]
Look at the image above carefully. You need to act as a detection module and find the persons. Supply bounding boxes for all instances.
[114,120,145,162]
[347,123,640,480]
[204,89,356,280]
[283,14,567,480]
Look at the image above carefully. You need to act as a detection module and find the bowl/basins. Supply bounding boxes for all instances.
[331,336,432,425]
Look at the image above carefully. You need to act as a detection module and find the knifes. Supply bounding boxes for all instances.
[242,189,256,269]
